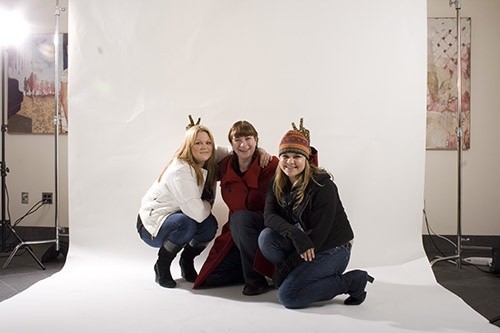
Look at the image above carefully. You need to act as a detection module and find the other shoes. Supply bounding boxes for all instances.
[243,281,274,296]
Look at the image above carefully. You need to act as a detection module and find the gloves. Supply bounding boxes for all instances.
[292,118,310,142]
[202,188,214,209]
[186,115,201,130]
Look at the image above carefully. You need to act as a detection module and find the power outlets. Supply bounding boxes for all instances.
[42,193,52,204]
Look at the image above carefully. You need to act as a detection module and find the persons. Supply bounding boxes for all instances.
[257,129,375,307]
[60,68,68,124]
[136,126,272,288]
[185,115,317,296]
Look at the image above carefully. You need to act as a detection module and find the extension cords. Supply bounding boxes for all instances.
[464,257,492,265]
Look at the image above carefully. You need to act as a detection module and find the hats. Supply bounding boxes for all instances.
[278,129,310,160]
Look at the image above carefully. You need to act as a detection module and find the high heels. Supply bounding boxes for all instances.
[344,274,374,305]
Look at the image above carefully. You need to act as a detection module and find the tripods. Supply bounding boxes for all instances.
[0,48,46,271]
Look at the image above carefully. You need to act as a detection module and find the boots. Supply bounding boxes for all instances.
[179,241,206,283]
[153,234,187,288]
